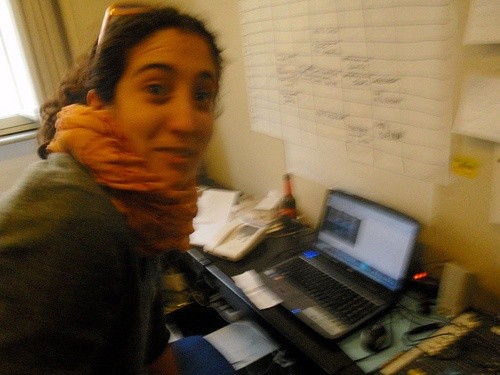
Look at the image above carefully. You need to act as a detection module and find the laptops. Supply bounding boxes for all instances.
[256,188,422,341]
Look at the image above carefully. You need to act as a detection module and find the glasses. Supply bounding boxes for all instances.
[92,4,150,65]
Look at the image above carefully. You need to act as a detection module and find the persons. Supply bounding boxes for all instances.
[0,7,239,375]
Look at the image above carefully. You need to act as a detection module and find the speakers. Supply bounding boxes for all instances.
[435,262,476,322]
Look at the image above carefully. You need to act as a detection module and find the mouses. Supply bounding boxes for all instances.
[365,325,386,351]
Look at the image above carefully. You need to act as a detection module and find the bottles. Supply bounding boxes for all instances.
[280,174,296,227]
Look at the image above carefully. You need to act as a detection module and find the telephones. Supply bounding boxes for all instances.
[202,217,268,264]
